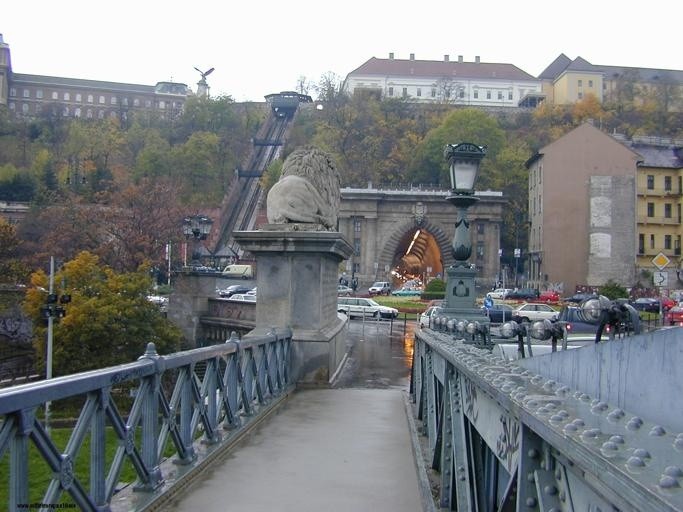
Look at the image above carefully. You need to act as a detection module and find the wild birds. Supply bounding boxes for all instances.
[193,66,216,79]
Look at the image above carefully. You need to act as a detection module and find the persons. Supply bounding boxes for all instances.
[339,272,348,287]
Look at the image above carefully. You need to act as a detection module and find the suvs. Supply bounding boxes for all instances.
[367,281,391,296]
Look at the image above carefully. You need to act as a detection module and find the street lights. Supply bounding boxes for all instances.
[44,254,72,432]
[183,213,214,266]
[534,256,543,301]
[443,142,488,306]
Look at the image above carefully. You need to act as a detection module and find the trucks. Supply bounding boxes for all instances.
[222,263,254,279]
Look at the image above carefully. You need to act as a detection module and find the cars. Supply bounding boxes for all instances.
[481,288,560,322]
[561,293,683,335]
[392,286,423,295]
[338,285,355,296]
[144,294,165,305]
[215,281,257,302]
[189,262,223,273]
[338,297,399,319]
